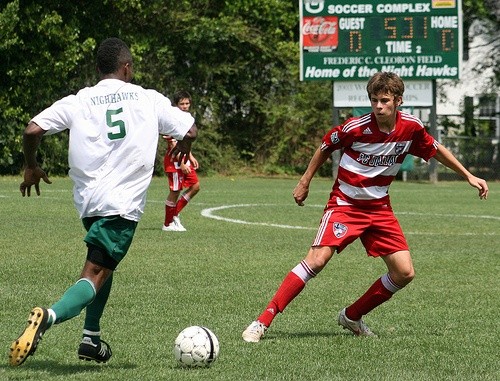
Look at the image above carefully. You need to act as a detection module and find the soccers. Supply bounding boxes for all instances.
[175,326,220,369]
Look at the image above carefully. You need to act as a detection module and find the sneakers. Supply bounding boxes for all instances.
[8,307,48,367]
[78,337,112,363]
[161,221,183,231]
[173,216,186,231]
[338,306,373,336]
[242,320,268,342]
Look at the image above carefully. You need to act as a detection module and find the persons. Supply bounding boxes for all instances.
[162,89,201,233]
[242,71,489,343]
[7,37,197,368]
